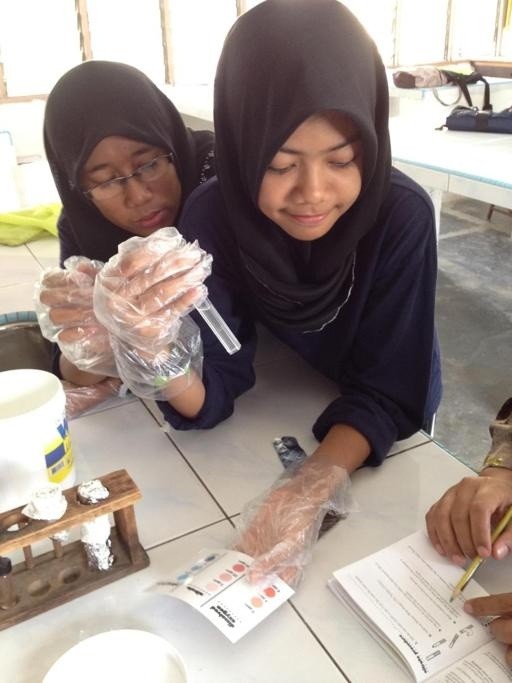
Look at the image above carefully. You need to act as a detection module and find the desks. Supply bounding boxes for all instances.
[0,233,512,683]
[178,69,512,245]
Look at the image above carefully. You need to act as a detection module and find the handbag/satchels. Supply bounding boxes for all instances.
[440,61,482,86]
[445,105,512,134]
[393,67,446,89]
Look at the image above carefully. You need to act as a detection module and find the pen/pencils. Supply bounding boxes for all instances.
[450,506,512,603]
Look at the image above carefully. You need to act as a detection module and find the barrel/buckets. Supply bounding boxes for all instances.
[0,368,77,515]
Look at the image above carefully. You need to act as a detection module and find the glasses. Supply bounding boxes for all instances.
[79,151,174,203]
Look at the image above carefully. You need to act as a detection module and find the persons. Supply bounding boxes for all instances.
[94,0,438,585]
[426,393,511,662]
[39,61,219,422]
